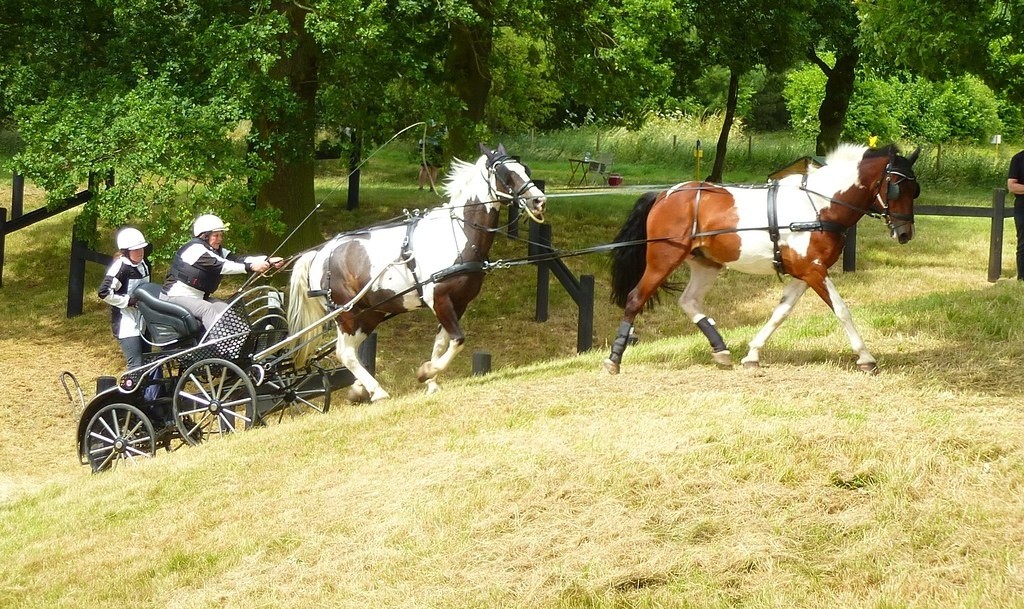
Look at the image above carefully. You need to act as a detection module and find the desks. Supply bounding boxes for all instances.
[567,159,592,187]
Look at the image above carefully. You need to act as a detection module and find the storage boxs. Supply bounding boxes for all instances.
[608,172,623,186]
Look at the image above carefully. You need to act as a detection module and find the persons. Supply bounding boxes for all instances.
[98,226,152,385]
[158,214,285,359]
[1007,149,1024,281]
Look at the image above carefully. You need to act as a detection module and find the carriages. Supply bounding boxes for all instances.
[60,142,921,474]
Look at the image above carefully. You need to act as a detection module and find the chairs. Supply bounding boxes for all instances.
[132,283,202,347]
[584,153,616,186]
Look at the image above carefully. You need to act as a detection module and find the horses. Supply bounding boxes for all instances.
[602,141,923,377]
[284,139,549,402]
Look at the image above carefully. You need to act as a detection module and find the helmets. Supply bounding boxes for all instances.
[194,215,227,236]
[116,227,148,251]
